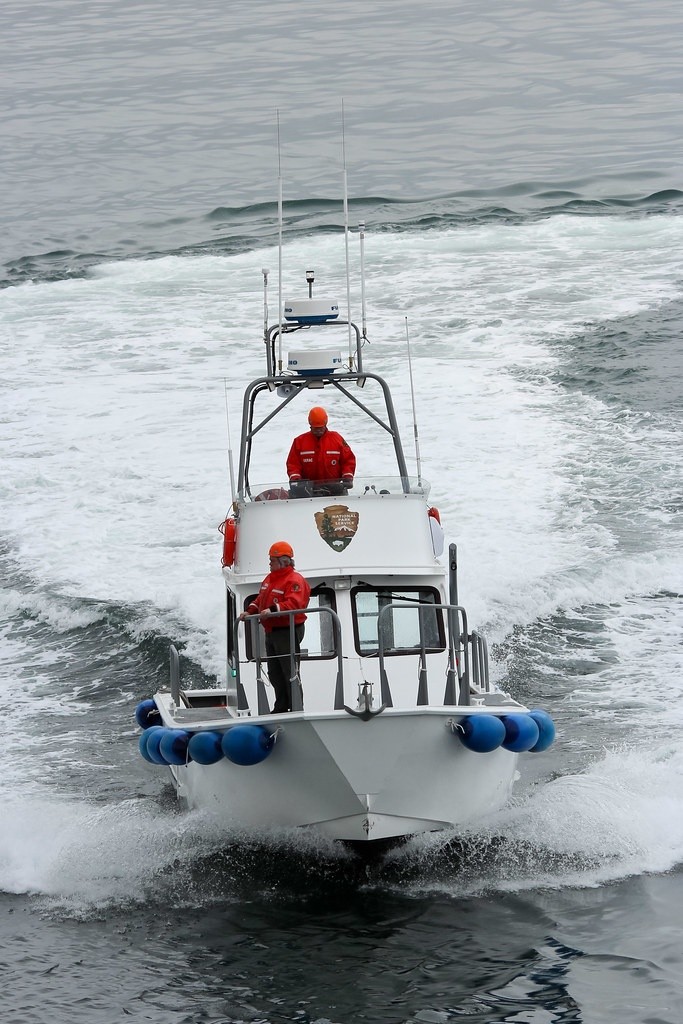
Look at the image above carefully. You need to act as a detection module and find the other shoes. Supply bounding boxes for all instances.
[270,705,289,714]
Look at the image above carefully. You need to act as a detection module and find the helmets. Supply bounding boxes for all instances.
[308,407,328,427]
[268,542,293,557]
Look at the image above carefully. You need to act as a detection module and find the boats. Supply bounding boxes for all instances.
[134,98,554,857]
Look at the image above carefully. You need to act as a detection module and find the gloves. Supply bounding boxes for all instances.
[339,478,353,489]
[291,481,298,491]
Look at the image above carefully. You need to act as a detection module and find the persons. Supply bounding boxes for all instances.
[286,407,356,489]
[240,541,310,713]
